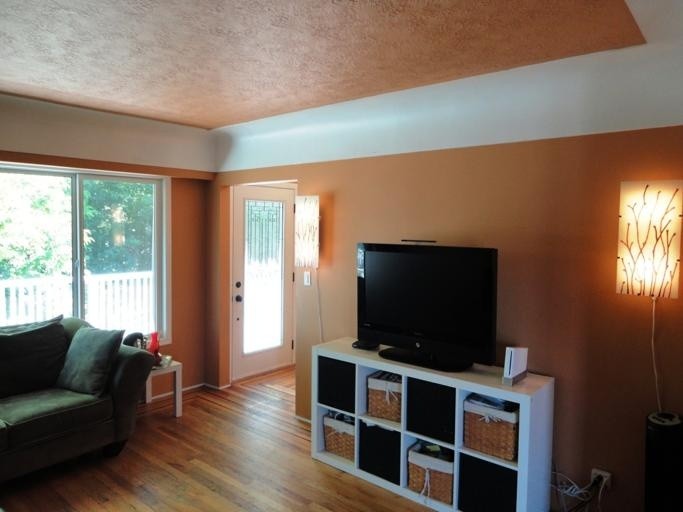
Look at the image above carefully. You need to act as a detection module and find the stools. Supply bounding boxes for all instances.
[142,351,183,418]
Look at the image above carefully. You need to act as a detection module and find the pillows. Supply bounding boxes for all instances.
[56,323,125,395]
[0,314,70,398]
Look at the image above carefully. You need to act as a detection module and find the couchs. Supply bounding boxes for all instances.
[0,319,154,494]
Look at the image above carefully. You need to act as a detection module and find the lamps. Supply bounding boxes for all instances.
[293,195,322,267]
[615,174,683,300]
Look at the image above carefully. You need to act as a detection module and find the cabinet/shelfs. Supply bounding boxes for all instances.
[311,336,554,512]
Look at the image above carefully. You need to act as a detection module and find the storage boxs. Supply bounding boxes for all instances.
[323,413,356,462]
[324,410,357,467]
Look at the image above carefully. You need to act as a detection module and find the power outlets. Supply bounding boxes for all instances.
[302,272,311,286]
[591,468,611,491]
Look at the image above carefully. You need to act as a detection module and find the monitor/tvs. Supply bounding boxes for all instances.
[356,243,498,374]
